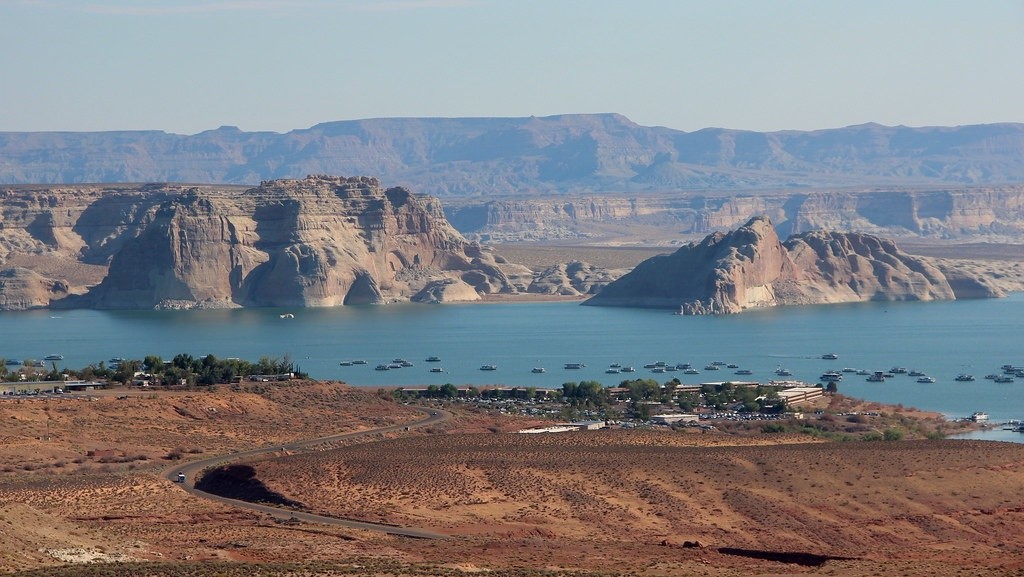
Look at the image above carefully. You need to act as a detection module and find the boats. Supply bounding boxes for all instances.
[109,357,126,369]
[339,361,353,366]
[6,359,44,366]
[564,363,586,369]
[45,354,64,360]
[985,365,1024,383]
[531,367,546,374]
[428,368,444,372]
[139,354,240,372]
[375,358,414,370]
[426,356,441,362]
[774,364,782,373]
[821,353,838,359]
[479,365,498,370]
[819,366,935,384]
[778,369,794,376]
[972,412,988,420]
[279,313,295,319]
[351,360,368,364]
[603,361,755,375]
[954,374,976,382]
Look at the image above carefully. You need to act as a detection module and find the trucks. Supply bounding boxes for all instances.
[56,389,63,394]
